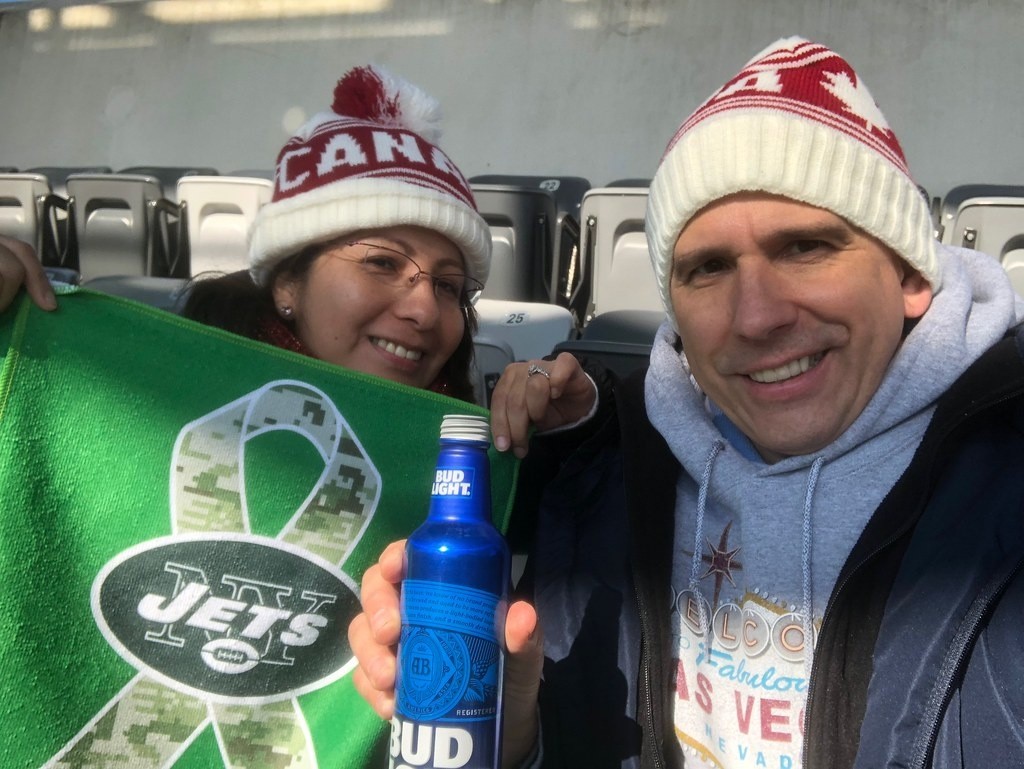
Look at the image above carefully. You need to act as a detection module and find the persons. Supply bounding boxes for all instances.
[0,66,650,769]
[346,37,1024,769]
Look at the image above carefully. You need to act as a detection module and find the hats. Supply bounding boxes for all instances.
[246,63,493,300]
[645,35,941,336]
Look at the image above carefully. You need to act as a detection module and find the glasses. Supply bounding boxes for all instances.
[324,241,484,309]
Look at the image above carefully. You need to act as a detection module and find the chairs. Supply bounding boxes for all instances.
[0,167,1024,587]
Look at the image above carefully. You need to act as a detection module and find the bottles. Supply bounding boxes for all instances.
[389,413,513,769]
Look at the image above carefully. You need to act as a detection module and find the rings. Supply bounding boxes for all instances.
[529,364,550,380]
